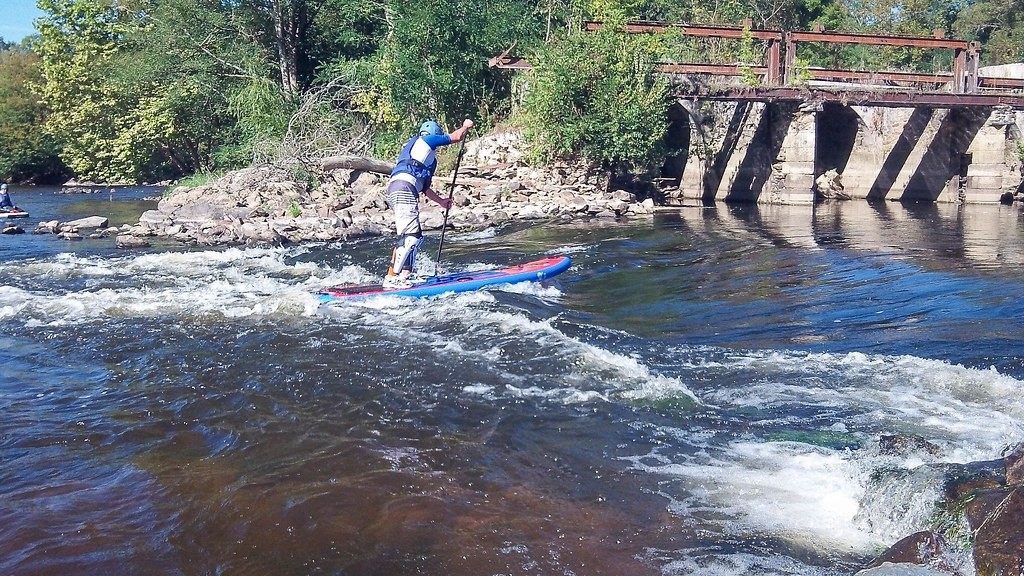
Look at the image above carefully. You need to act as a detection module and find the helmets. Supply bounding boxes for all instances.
[1,184,8,189]
[419,121,444,136]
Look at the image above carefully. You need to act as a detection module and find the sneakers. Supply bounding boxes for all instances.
[382,275,413,288]
[399,272,426,284]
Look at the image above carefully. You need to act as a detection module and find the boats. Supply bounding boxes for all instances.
[0,206,30,218]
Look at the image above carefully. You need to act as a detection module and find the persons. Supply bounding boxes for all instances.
[381,119,475,288]
[0,184,24,213]
